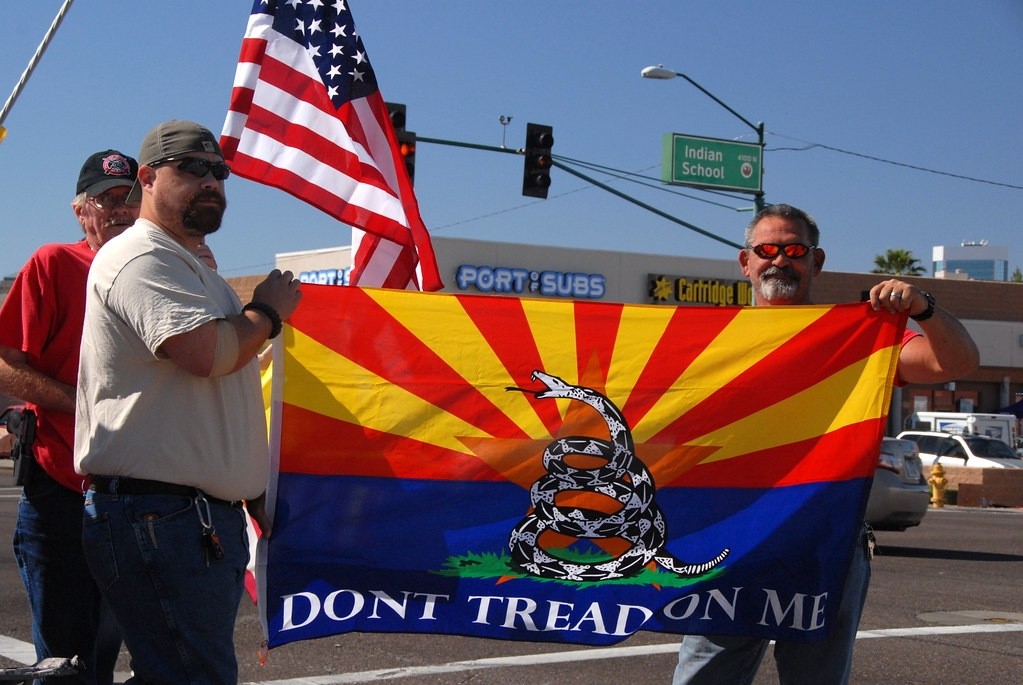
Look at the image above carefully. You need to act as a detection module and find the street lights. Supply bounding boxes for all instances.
[640,66,765,219]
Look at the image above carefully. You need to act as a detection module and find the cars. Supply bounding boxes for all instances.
[0,404,26,458]
[863,437,931,531]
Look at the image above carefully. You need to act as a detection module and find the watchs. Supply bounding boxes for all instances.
[909,288,936,322]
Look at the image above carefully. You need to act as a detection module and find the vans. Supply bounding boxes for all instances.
[903,411,1019,451]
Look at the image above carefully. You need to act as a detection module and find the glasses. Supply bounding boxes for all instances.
[82,193,142,209]
[748,243,816,259]
[147,157,230,181]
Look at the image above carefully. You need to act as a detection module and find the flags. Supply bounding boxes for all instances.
[220,0,445,293]
[253,282,908,650]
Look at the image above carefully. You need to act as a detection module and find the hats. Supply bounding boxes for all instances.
[76,150,139,197]
[125,120,224,205]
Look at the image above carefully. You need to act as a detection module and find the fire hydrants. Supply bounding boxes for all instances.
[925,461,950,508]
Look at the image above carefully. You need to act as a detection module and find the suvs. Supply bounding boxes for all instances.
[895,430,1023,472]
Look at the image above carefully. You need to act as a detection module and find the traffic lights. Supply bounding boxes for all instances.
[385,102,408,136]
[396,131,417,186]
[520,120,555,200]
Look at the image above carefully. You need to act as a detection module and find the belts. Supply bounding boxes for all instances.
[90,475,244,507]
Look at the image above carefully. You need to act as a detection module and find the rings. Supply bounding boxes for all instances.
[890,291,901,298]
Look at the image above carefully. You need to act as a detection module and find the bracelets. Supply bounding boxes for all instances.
[241,301,282,338]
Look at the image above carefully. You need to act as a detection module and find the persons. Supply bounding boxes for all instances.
[1,150,217,662]
[72,120,301,685]
[670,203,979,685]
